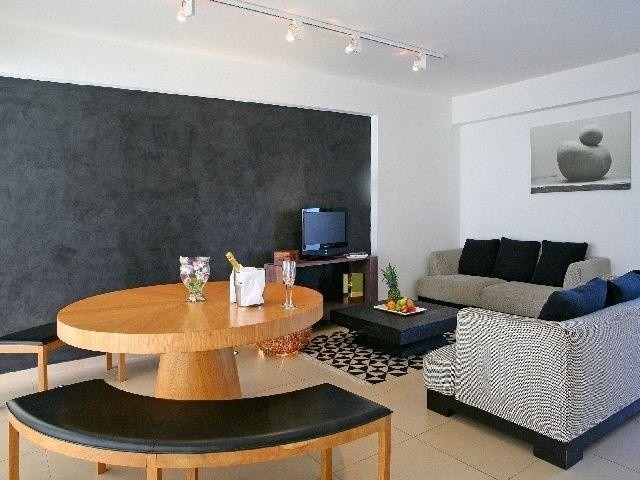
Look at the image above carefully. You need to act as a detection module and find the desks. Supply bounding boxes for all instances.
[56,280,323,399]
[264,255,378,307]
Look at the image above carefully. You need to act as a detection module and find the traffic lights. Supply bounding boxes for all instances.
[462,237,587,287]
[531,271,639,320]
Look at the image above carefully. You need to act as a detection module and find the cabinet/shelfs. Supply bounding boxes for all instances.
[226,251,240,272]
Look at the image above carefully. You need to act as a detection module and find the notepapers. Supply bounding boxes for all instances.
[379,263,400,301]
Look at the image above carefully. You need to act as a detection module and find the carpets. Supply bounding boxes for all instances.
[296,326,456,388]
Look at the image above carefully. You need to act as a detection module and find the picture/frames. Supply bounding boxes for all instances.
[264,255,378,307]
[56,280,323,399]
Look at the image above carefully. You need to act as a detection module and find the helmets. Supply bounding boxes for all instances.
[373,301,427,317]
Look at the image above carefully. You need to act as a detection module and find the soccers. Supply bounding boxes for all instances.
[397,298,408,306]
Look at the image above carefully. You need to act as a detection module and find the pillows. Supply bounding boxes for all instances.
[531,271,639,320]
[462,237,587,287]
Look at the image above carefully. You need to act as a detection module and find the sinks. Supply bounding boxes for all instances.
[423,297,639,468]
[412,241,613,319]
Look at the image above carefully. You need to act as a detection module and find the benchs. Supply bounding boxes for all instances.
[5,379,395,480]
[2,322,113,392]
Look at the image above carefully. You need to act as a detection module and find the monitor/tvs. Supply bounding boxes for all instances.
[349,252,369,256]
[343,253,367,258]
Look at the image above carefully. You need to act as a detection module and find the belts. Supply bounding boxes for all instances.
[280,261,297,308]
[179,256,211,303]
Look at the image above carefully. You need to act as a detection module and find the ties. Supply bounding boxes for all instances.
[301,208,349,261]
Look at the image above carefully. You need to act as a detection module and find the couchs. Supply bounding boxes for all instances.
[412,241,613,319]
[423,297,639,468]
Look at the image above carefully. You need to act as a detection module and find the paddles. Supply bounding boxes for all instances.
[296,326,456,388]
